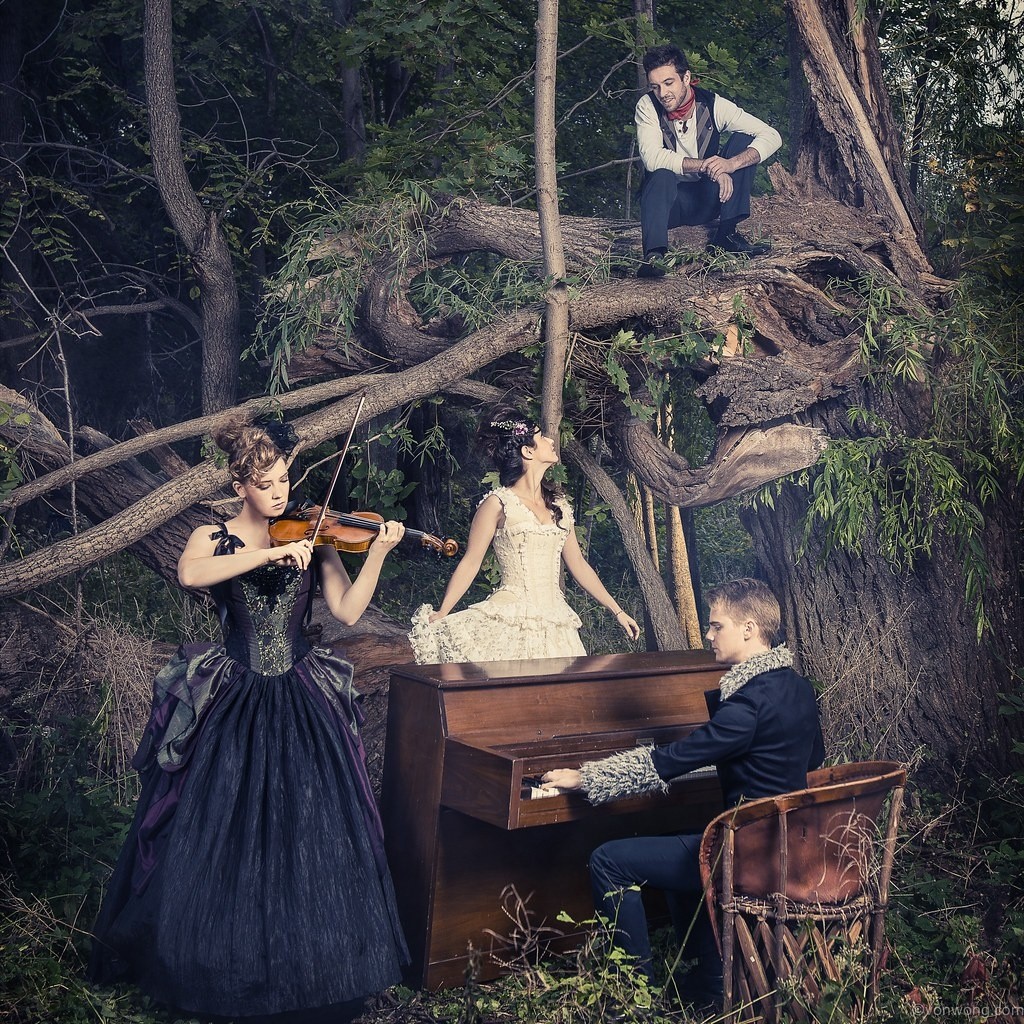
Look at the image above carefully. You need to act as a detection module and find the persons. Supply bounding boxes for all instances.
[83,425,412,1024]
[404,410,643,668]
[535,576,825,992]
[635,44,784,279]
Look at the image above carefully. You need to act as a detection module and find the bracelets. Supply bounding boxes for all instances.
[612,609,625,619]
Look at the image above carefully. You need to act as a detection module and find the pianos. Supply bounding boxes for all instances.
[381,650,732,997]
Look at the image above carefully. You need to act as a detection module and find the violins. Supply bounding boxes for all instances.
[268,497,458,557]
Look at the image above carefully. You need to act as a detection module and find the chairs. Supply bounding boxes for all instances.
[699,762,907,1024]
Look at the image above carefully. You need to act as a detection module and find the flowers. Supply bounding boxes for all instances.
[491,420,529,435]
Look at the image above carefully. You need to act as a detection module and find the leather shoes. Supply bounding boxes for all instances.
[683,965,733,1003]
[714,231,771,255]
[574,994,663,1024]
[642,254,666,277]
